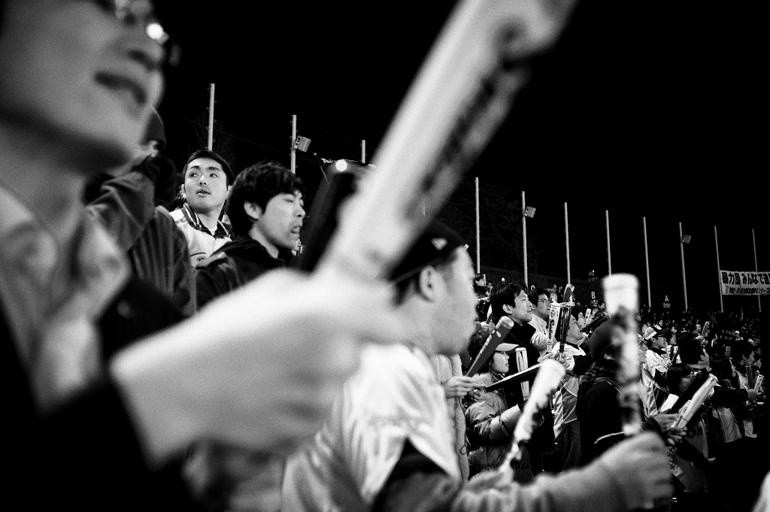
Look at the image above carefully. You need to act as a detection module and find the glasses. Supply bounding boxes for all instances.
[94,0,139,26]
[538,298,552,303]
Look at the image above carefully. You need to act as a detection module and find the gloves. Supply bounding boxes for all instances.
[131,155,175,202]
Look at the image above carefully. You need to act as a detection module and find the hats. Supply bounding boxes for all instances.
[468,329,519,351]
[391,219,465,282]
[144,109,166,149]
[643,324,662,340]
[588,320,611,357]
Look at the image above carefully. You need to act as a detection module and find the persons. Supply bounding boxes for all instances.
[0,0,426,508]
[85,104,770,511]
